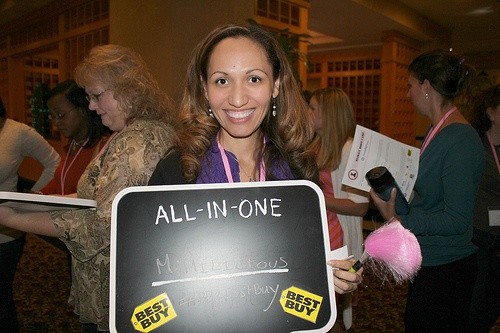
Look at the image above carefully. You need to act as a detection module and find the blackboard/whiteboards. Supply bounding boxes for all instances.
[109,180,338,333]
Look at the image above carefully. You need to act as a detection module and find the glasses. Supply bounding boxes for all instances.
[48,107,77,120]
[85,87,110,102]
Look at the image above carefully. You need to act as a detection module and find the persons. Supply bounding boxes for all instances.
[0,43,181,333]
[473,83,500,240]
[369,49,489,333]
[27,80,113,196]
[305,86,364,333]
[147,23,365,333]
[0,101,61,333]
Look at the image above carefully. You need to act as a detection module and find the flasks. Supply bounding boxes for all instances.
[365,166,412,216]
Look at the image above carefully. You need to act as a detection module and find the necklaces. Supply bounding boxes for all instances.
[234,159,257,183]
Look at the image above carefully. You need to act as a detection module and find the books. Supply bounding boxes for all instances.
[2,191,100,213]
